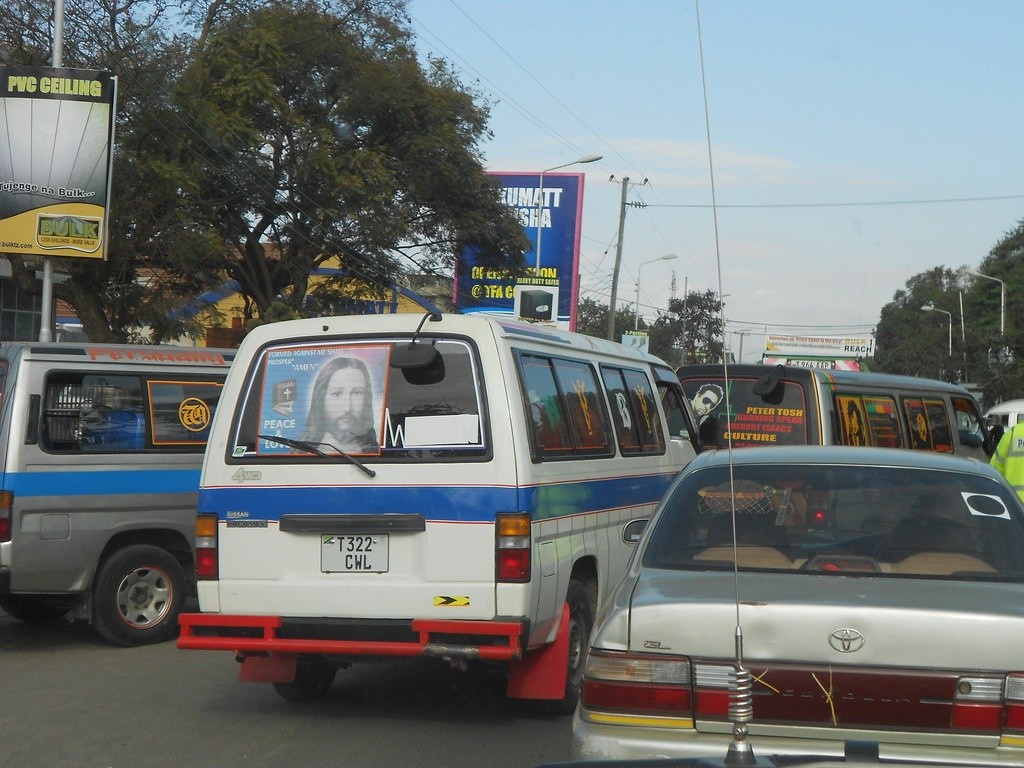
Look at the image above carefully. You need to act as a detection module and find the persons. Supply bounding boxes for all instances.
[74,385,137,451]
[878,493,972,563]
[688,384,723,431]
[983,420,1024,504]
[289,357,379,453]
[847,400,866,446]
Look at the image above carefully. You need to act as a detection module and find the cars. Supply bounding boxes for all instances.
[575,447,1022,766]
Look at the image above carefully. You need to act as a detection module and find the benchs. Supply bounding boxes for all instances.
[693,543,997,575]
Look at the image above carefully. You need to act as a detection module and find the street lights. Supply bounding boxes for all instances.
[537,154,606,279]
[920,305,953,355]
[633,252,675,330]
[966,268,1005,347]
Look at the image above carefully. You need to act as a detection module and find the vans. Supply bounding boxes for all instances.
[173,311,698,716]
[969,399,1024,449]
[0,344,272,646]
[660,362,1004,477]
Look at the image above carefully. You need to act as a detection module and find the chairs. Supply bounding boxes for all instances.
[878,516,977,564]
[706,510,793,558]
